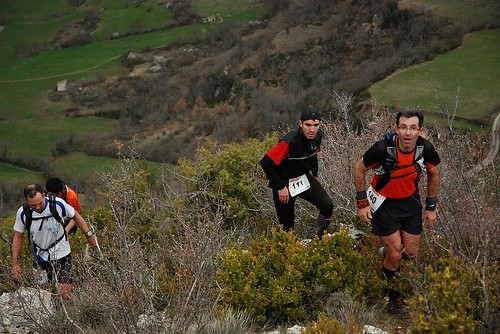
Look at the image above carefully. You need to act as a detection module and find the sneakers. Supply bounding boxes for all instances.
[378,247,385,261]
[381,289,408,319]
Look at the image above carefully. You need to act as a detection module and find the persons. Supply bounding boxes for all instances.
[41,177,82,240]
[10,182,98,306]
[258,107,334,242]
[354,109,441,318]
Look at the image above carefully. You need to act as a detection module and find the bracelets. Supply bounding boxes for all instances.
[425,196,438,211]
[85,229,93,237]
[356,191,369,209]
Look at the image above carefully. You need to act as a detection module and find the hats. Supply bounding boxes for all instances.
[301,106,321,121]
